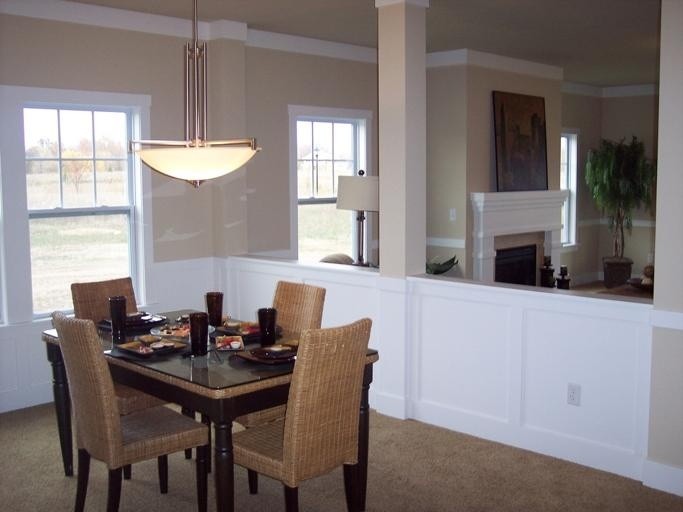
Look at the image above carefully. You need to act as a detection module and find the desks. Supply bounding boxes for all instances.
[42,307,380,512]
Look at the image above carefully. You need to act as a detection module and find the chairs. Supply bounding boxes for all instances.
[269,280,328,336]
[71,279,139,321]
[50,311,212,512]
[228,317,373,512]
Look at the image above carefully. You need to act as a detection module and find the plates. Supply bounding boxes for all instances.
[214,335,246,352]
[149,322,217,338]
[114,334,188,359]
[95,312,170,332]
[627,277,653,290]
[214,324,284,339]
[233,344,298,365]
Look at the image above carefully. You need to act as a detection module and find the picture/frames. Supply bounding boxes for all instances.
[493,90,548,192]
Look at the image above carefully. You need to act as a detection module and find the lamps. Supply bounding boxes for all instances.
[336,170,379,266]
[129,0,262,187]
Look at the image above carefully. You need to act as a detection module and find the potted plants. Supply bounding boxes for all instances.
[585,135,656,289]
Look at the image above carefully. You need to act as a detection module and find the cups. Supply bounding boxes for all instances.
[108,296,127,345]
[189,312,209,356]
[206,291,223,328]
[258,307,277,346]
